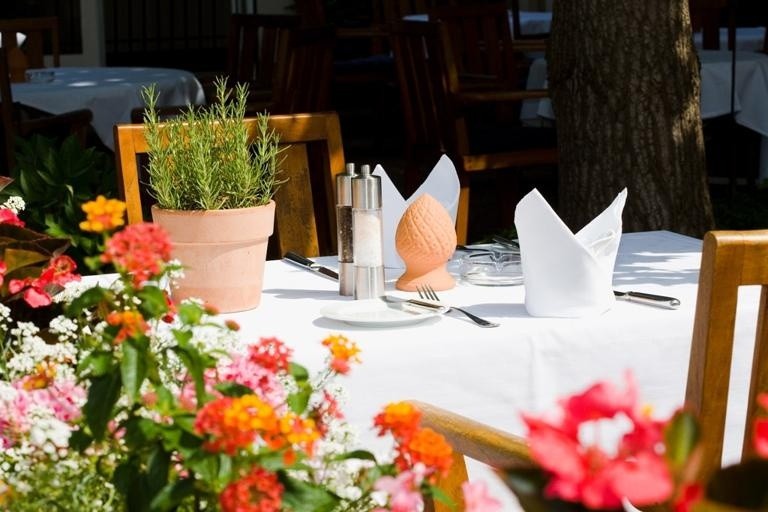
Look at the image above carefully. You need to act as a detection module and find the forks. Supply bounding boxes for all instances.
[417,283,502,328]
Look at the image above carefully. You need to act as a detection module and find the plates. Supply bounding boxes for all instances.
[322,296,451,327]
[459,257,525,287]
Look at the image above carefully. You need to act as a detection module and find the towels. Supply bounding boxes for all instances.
[369,151,463,270]
[513,186,630,321]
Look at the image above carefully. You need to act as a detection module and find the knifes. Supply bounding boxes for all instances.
[614,290,682,311]
[282,250,338,282]
[381,295,445,313]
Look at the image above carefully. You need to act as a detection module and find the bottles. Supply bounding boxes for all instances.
[334,162,361,297]
[350,164,386,299]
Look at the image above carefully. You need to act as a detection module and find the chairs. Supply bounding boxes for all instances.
[111,109,346,267]
[387,226,768,512]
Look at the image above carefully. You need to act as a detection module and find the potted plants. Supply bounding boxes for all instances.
[139,72,291,316]
